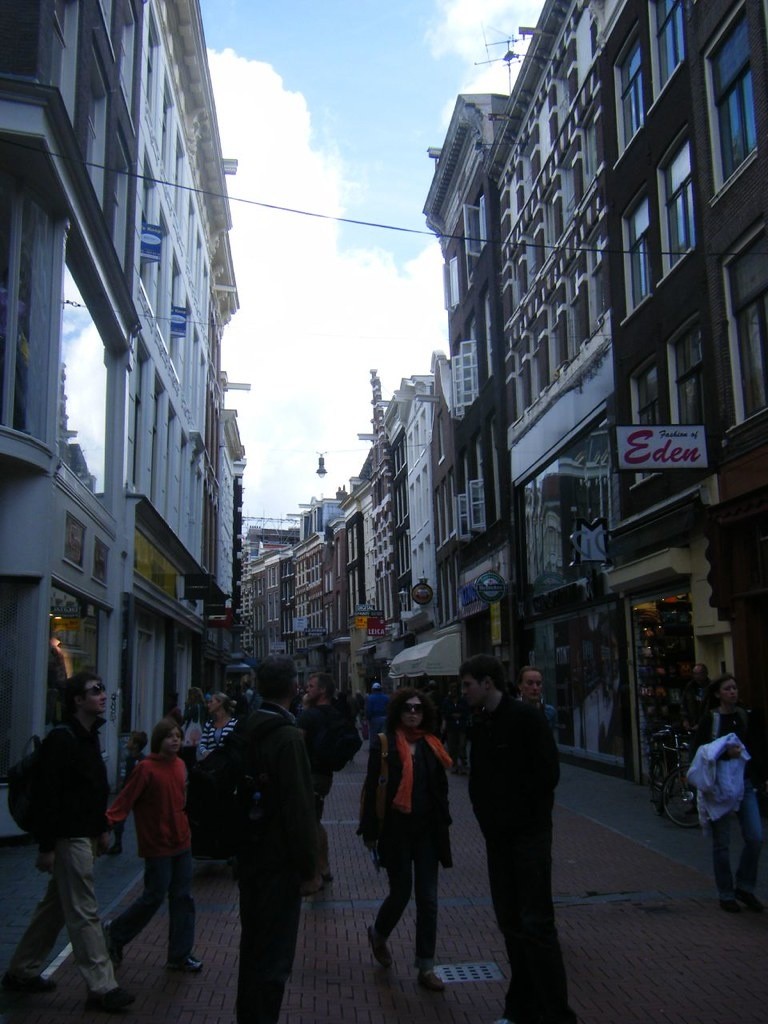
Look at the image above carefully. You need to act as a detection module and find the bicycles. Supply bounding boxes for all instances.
[646,725,710,828]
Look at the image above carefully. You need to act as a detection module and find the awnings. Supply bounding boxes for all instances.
[386,630,461,678]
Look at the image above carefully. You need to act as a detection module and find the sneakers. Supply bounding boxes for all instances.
[167,955,202,971]
[101,919,123,963]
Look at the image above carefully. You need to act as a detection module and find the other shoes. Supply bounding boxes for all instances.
[2,970,56,992]
[719,899,739,912]
[418,971,444,990]
[301,884,324,896]
[369,923,393,968]
[734,888,763,912]
[321,872,333,881]
[87,987,135,1008]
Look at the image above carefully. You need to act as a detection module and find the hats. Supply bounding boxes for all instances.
[372,683,382,689]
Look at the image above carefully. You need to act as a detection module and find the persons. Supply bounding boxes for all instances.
[1,673,137,1008]
[232,655,324,1024]
[456,655,578,1024]
[166,674,263,878]
[291,673,333,897]
[356,684,453,994]
[105,718,203,972]
[514,665,559,747]
[680,663,764,913]
[337,679,480,773]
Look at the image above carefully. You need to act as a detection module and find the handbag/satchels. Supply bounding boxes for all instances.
[180,704,203,747]
[360,733,389,826]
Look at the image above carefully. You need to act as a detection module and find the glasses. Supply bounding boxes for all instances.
[82,684,105,693]
[400,703,425,714]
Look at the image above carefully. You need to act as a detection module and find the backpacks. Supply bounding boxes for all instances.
[307,708,362,772]
[183,710,296,849]
[8,724,74,832]
